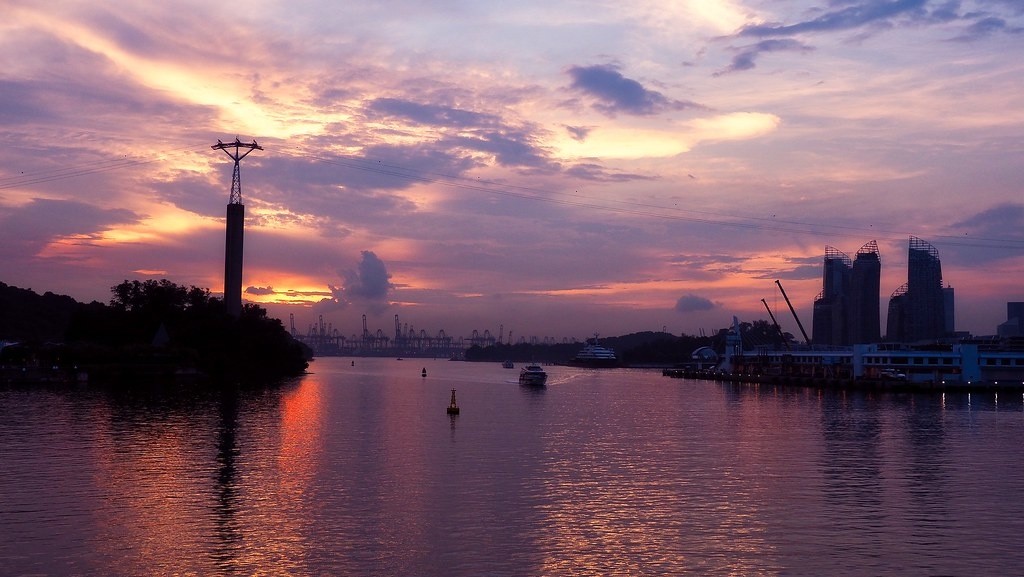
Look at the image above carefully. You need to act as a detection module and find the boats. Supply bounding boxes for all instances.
[519,363,548,386]
[397,359,403,360]
[503,360,514,369]
[576,328,617,367]
